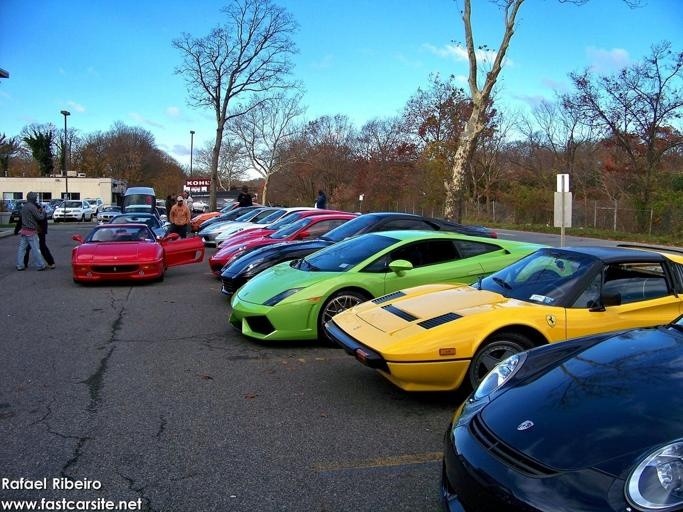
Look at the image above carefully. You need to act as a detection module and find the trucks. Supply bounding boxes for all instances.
[122,188,155,213]
[66,171,86,178]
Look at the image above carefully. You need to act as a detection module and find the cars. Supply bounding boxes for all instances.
[109,213,170,238]
[96,206,120,221]
[53,200,91,222]
[71,224,204,283]
[155,199,224,214]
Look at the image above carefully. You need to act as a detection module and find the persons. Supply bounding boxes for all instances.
[15,191,47,271]
[314,189,326,209]
[165,189,193,241]
[238,185,252,207]
[14,203,57,269]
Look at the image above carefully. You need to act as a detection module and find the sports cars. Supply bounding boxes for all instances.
[439,313,682,496]
[220,211,496,295]
[324,245,682,392]
[192,202,363,279]
[227,230,554,345]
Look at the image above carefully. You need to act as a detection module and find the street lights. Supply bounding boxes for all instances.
[189,131,195,178]
[60,110,70,198]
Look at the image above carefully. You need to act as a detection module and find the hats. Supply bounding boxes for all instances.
[177,196,183,201]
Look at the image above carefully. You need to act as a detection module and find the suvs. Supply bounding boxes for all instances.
[0,199,61,219]
[83,198,103,215]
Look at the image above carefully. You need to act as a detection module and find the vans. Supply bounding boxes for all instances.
[124,205,151,213]
